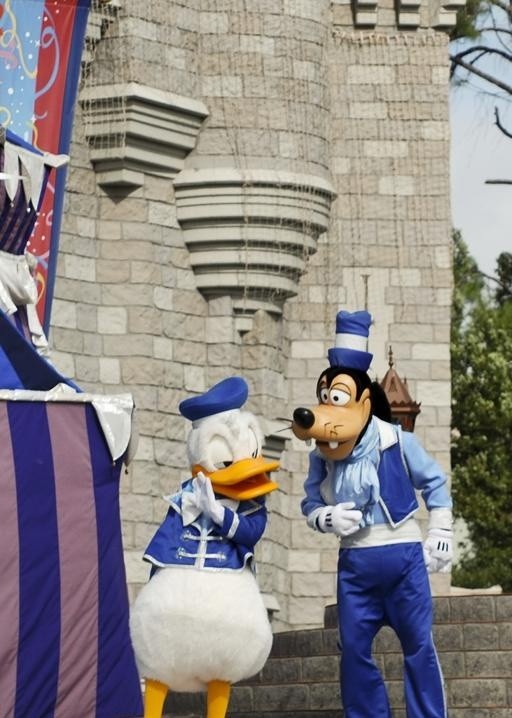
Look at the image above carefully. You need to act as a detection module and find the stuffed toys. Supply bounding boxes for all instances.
[130,376,281,718]
[290,311,455,718]
